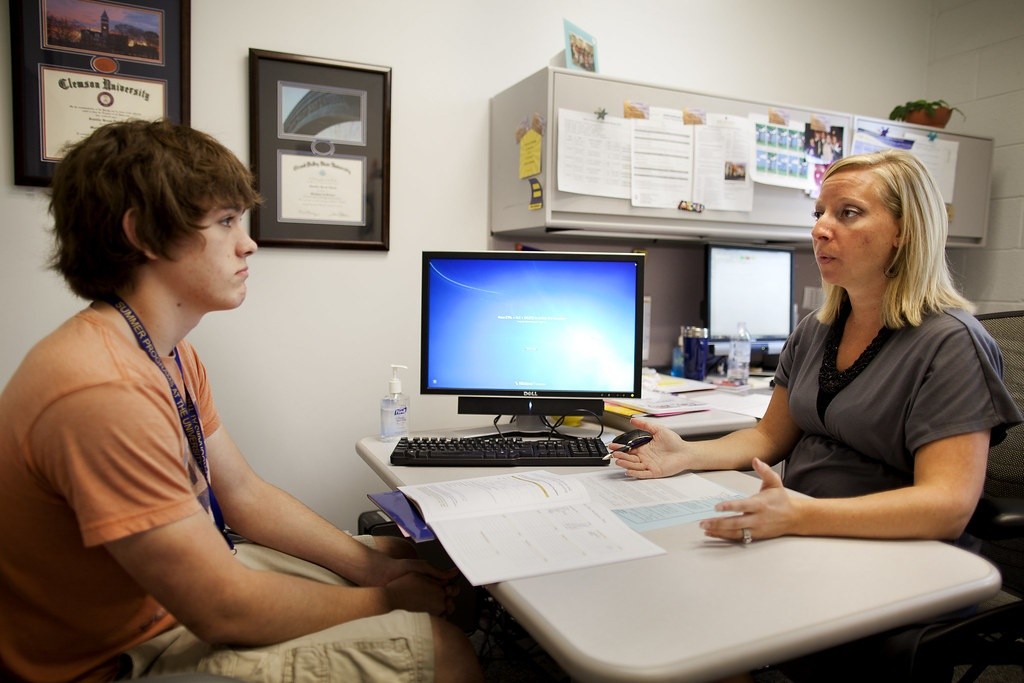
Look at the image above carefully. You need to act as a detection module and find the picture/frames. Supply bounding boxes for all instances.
[249,47,393,252]
[8,0,190,189]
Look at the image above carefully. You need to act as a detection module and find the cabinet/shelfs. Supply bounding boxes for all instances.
[487,68,994,250]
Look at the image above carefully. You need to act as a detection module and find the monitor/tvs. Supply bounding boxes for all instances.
[420,250,647,399]
[702,244,795,356]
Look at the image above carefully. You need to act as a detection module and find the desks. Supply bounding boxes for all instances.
[583,371,779,435]
[357,418,1002,683]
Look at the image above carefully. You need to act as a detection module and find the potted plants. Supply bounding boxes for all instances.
[887,99,967,129]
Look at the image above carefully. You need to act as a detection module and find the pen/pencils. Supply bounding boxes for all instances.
[601,437,653,461]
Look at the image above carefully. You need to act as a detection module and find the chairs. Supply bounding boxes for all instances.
[894,310,1024,679]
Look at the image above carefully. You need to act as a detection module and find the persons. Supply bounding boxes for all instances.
[570,34,595,71]
[0,122,484,683]
[604,149,1017,683]
[806,131,840,161]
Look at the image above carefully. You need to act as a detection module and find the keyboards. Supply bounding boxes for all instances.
[389,436,611,468]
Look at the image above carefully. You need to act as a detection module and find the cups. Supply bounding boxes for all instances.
[680,326,709,382]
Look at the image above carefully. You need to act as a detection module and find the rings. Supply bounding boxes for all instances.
[740,527,753,545]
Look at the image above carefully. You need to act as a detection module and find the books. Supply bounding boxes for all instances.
[603,391,709,420]
[392,469,670,588]
[364,490,437,544]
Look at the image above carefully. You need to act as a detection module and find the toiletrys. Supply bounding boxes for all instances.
[669,323,709,381]
[380,363,412,445]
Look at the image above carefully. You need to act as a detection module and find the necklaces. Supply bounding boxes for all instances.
[109,292,236,555]
[819,300,905,393]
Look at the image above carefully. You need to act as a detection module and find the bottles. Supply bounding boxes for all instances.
[670,335,685,377]
[727,321,753,387]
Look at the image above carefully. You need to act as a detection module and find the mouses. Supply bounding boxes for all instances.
[612,429,653,450]
